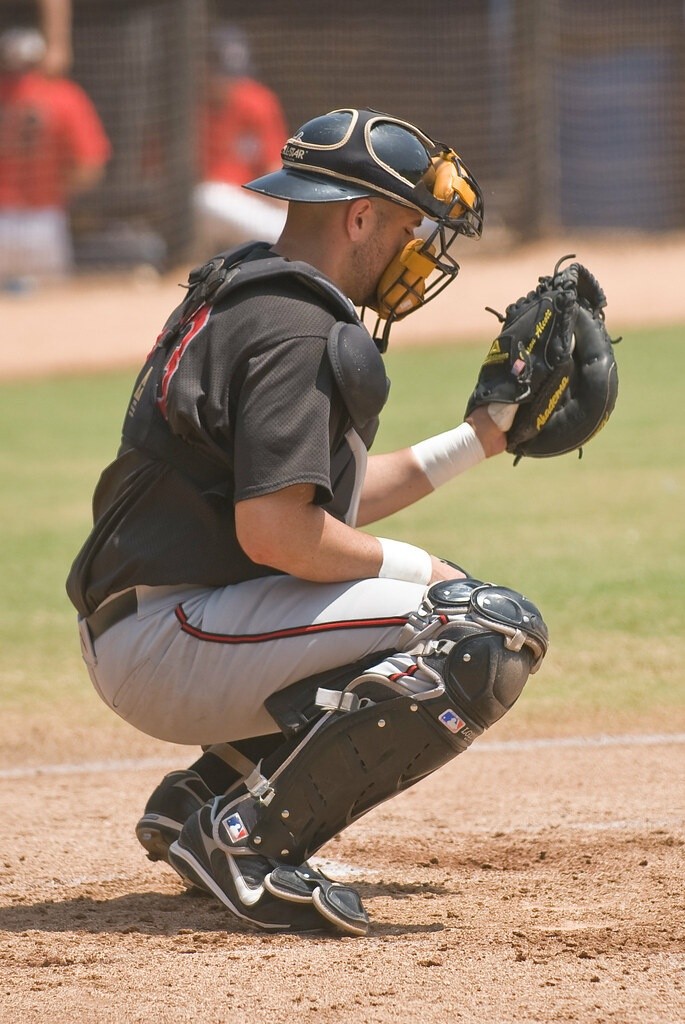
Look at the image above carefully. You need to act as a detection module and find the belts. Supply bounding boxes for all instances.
[83,588,138,645]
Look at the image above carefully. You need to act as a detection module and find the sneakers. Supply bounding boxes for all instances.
[135,768,217,896]
[167,796,338,934]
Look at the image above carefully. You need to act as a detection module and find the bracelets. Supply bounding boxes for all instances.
[411,421,488,491]
[373,536,432,586]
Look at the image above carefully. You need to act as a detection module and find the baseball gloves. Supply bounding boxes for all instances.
[462,262,620,459]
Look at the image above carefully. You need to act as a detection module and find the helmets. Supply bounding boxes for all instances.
[240,105,485,241]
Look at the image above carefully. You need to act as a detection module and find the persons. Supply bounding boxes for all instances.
[188,31,292,245]
[0,27,115,279]
[65,106,622,934]
